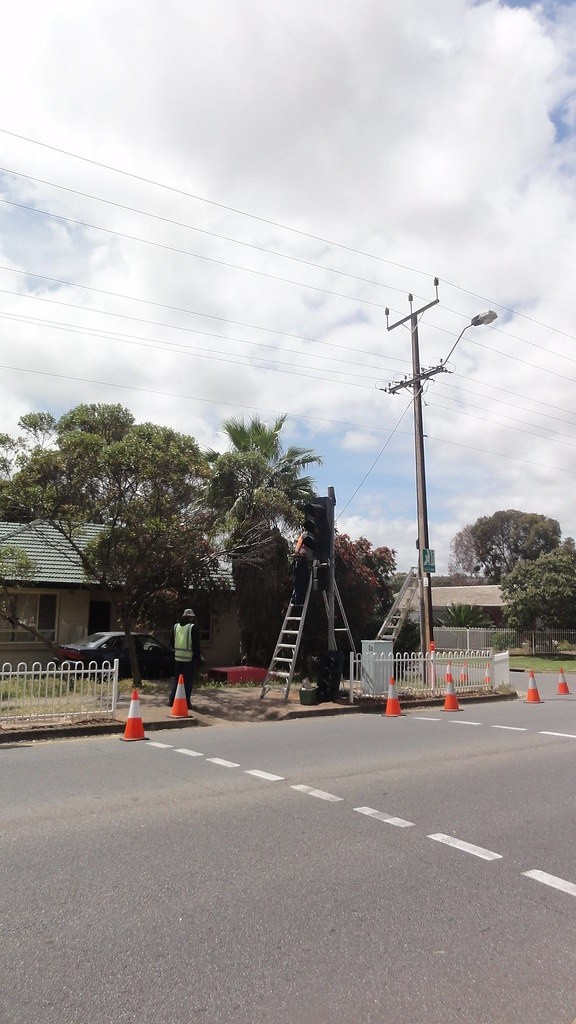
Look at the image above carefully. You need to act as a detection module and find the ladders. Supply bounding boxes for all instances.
[259,560,313,703]
[320,561,361,693]
[375,566,420,648]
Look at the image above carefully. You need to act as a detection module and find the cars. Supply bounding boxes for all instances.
[52,629,207,682]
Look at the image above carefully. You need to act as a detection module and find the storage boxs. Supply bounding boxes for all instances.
[299,685,320,705]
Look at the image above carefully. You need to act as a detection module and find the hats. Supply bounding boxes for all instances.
[182,609,195,616]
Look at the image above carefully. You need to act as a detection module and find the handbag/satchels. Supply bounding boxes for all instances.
[292,553,308,571]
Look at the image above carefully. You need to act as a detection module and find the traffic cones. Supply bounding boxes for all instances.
[523,667,545,704]
[119,689,150,742]
[556,667,572,695]
[440,672,464,712]
[445,660,453,676]
[460,660,469,681]
[381,676,407,717]
[166,674,194,718]
[483,662,491,685]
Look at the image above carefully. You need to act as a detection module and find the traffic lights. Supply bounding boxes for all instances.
[303,496,329,559]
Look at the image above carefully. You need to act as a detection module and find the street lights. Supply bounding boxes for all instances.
[411,309,499,687]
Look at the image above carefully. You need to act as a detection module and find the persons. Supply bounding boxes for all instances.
[167,609,201,709]
[292,520,320,604]
